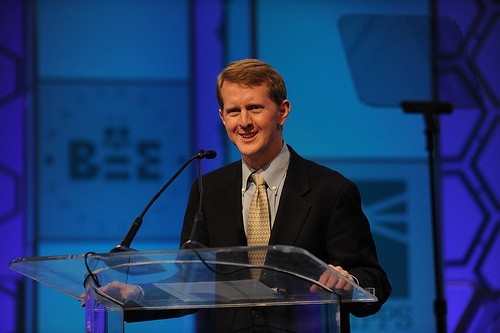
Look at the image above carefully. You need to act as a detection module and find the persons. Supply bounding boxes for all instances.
[82,59,392,333]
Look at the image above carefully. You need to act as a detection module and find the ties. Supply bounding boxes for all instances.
[246,173,272,281]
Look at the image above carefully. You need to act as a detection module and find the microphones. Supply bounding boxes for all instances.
[104,151,217,275]
[181,151,212,272]
